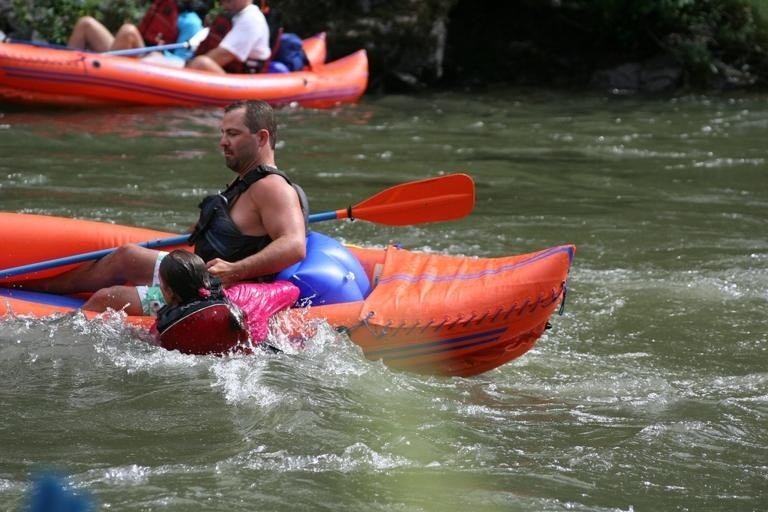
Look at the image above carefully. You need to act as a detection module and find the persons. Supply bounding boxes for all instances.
[0,96,310,315]
[131,248,301,360]
[66,0,281,73]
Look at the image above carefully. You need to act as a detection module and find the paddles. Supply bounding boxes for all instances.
[101,28,211,55]
[1,173,475,278]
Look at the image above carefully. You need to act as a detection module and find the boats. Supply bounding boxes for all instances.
[0,210,575,383]
[1,31,372,111]
